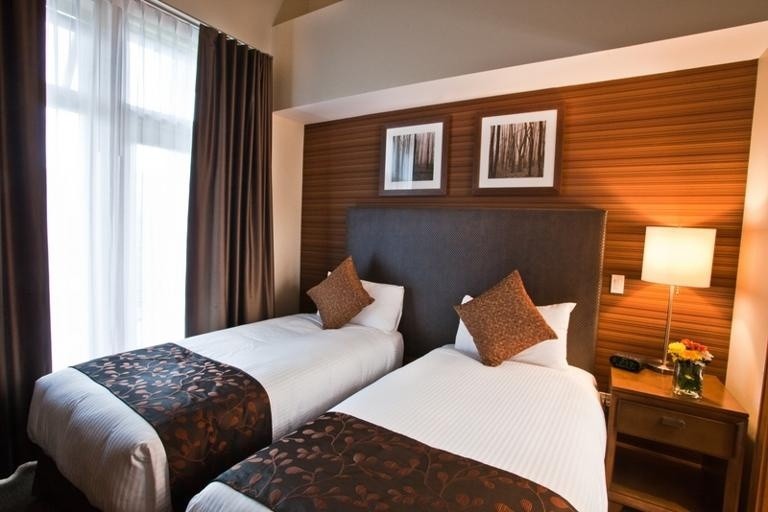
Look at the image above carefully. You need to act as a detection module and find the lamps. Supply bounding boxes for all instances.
[641,225,717,374]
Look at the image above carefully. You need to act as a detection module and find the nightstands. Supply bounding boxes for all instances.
[604,362,750,512]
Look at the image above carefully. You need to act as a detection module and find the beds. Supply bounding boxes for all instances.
[26,314,405,510]
[186,344,608,511]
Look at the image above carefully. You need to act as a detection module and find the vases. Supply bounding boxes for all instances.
[672,361,703,399]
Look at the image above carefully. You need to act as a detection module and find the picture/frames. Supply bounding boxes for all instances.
[378,114,452,197]
[472,102,564,197]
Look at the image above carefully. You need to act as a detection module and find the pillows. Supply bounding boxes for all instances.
[306,256,376,329]
[452,269,557,367]
[316,271,405,336]
[454,293,577,369]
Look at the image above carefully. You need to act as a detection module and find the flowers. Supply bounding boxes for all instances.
[668,338,714,392]
